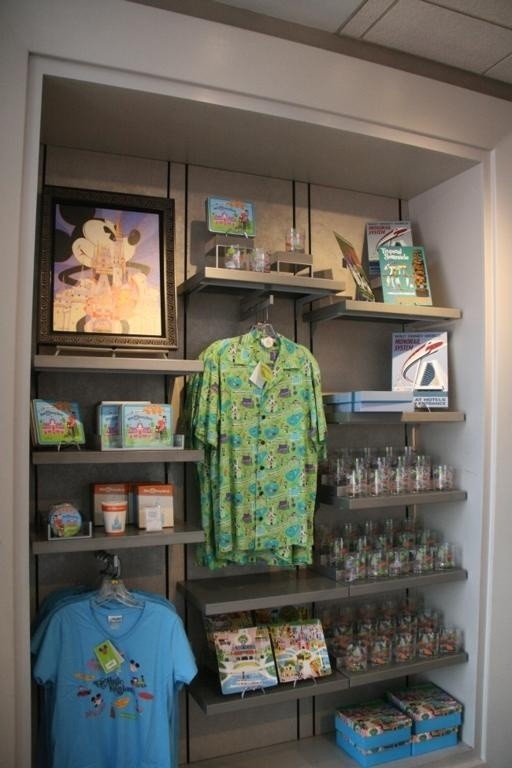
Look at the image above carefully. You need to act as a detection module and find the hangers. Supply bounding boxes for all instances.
[246,303,279,339]
[78,549,148,620]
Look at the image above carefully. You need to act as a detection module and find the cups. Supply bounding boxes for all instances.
[251,247,272,273]
[322,443,468,673]
[225,240,248,269]
[286,228,307,254]
[101,500,128,535]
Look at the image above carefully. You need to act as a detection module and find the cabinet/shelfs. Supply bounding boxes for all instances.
[29,264,470,768]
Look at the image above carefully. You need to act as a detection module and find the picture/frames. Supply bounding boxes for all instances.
[37,183,180,350]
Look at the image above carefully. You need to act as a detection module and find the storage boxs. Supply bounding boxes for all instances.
[334,680,463,767]
